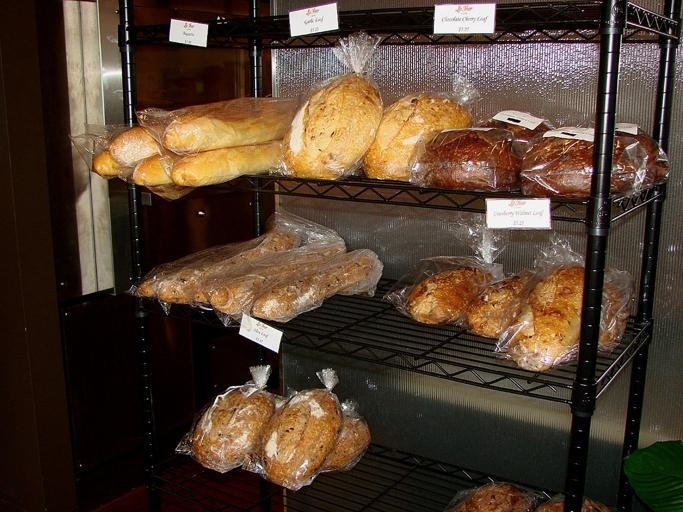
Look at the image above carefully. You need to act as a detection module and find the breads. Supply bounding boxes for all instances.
[450,483,532,512]
[534,496,609,512]
[407,266,630,371]
[92,73,670,201]
[138,232,375,321]
[190,386,371,485]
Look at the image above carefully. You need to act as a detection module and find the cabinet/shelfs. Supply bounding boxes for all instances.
[113,1,682,512]
